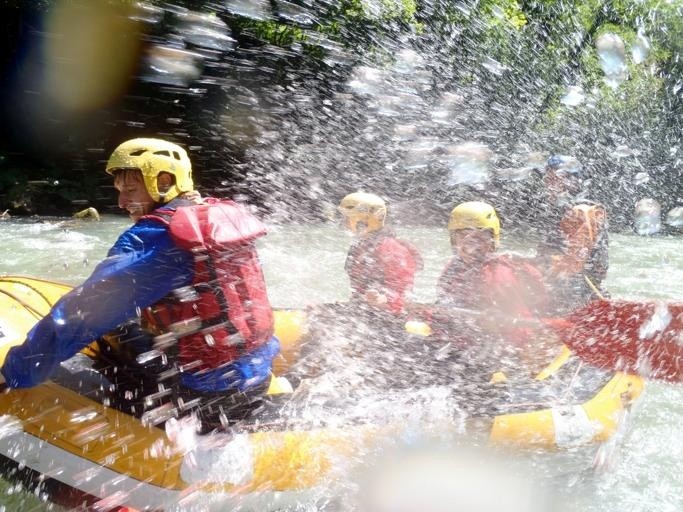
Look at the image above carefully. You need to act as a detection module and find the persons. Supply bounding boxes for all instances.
[287,155,609,415]
[0,137,280,434]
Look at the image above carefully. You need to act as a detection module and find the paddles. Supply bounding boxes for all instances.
[448,302,683,383]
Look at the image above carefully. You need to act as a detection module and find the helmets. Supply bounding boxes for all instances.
[337,190,383,225]
[104,135,193,204]
[444,201,501,251]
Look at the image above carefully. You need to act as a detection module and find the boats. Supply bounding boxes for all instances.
[0,276,646,512]
